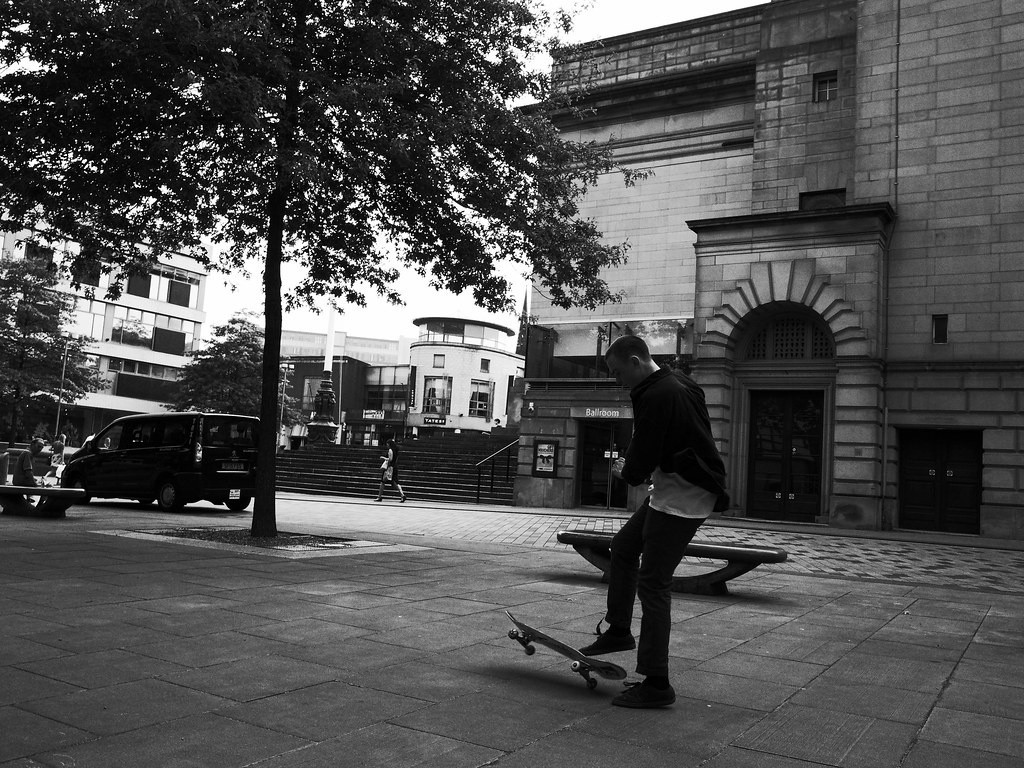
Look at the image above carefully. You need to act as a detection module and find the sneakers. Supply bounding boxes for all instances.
[578,618,636,656]
[612,681,676,707]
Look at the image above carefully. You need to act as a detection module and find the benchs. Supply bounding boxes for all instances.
[557,530,787,596]
[0,485,85,520]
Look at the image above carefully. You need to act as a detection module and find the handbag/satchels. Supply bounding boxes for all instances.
[380,458,389,470]
[382,466,393,482]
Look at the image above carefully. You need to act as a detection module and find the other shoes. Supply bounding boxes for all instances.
[400,494,406,503]
[374,498,382,502]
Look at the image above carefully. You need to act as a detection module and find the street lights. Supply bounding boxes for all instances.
[55,341,80,440]
[278,363,294,446]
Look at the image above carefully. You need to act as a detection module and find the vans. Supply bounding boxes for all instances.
[61,412,260,512]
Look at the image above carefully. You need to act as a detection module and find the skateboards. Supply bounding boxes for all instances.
[503,609,627,689]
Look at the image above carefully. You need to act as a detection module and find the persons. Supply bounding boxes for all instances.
[81,432,96,448]
[12,438,45,487]
[482,418,504,436]
[375,439,407,504]
[103,434,111,449]
[42,434,66,485]
[578,335,725,709]
